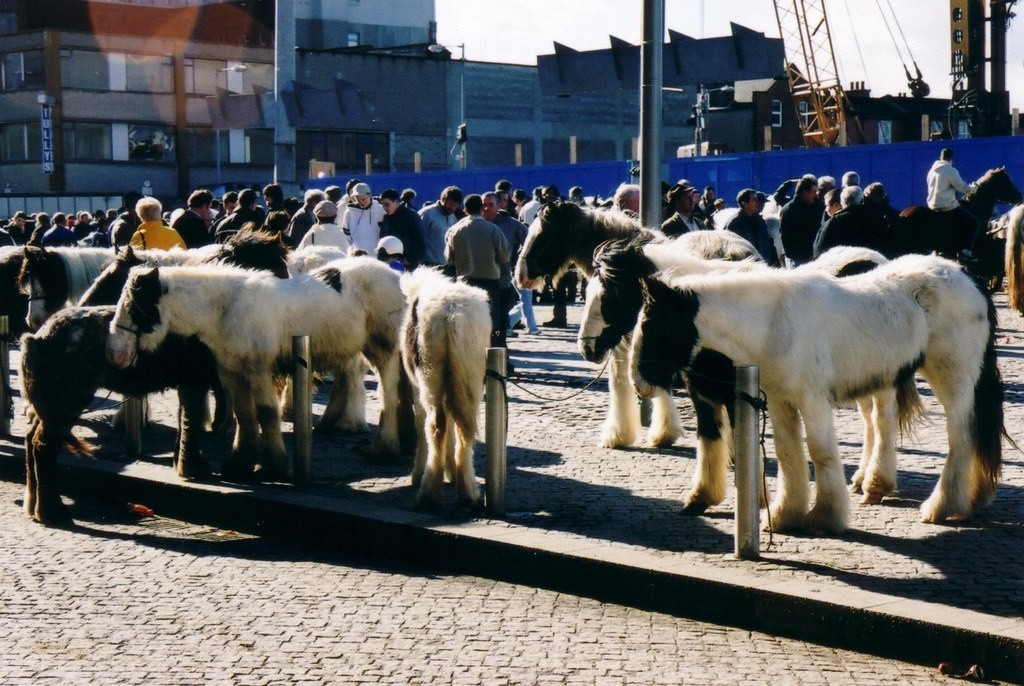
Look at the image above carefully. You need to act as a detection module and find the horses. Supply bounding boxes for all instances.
[512,164,1024,538]
[0,219,493,528]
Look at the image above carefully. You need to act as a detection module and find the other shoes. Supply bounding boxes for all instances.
[543,318,566,327]
[507,332,518,336]
[529,329,541,334]
[513,324,526,329]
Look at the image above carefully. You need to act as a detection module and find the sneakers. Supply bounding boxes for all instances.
[957,249,978,261]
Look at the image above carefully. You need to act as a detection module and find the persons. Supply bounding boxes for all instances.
[926,148,981,261]
[0,171,891,378]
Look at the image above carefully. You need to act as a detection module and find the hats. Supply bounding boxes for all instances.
[667,184,694,203]
[313,201,337,217]
[376,235,404,255]
[756,192,769,203]
[863,182,885,202]
[52,212,65,224]
[350,183,370,196]
[677,179,689,185]
[94,210,105,215]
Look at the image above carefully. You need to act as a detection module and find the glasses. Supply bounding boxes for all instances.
[482,202,496,206]
[226,206,235,211]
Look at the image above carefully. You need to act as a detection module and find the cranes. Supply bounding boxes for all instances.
[770,0,930,148]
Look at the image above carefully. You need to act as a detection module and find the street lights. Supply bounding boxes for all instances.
[215,66,248,181]
[429,43,468,169]
